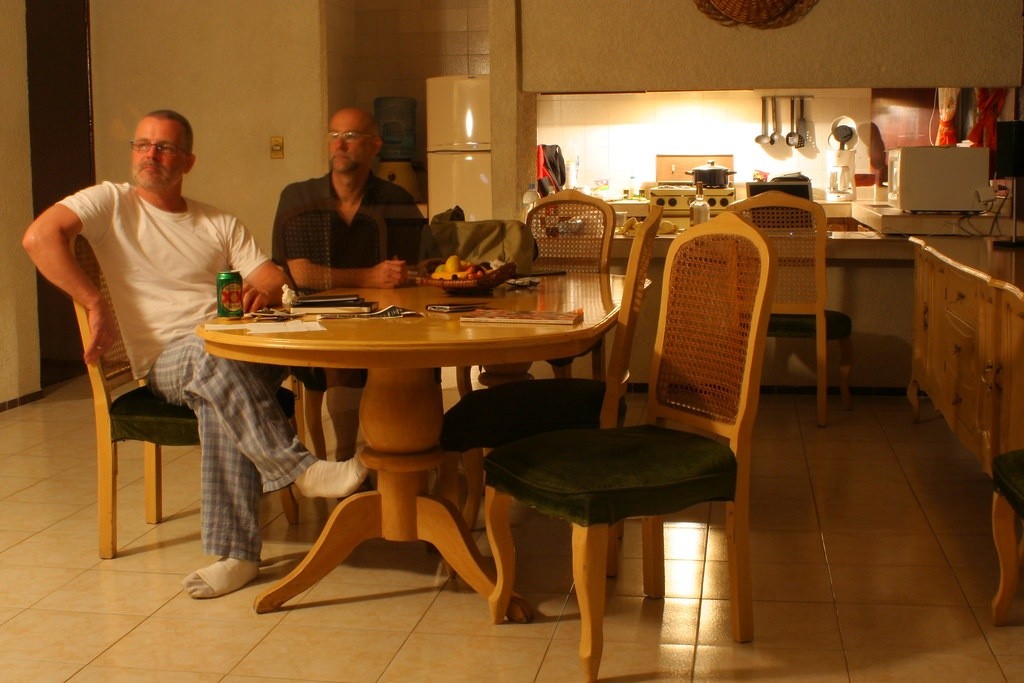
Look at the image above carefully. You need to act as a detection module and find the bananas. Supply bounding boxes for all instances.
[431,267,474,280]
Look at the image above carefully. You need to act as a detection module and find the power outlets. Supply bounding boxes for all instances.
[990,180,1014,199]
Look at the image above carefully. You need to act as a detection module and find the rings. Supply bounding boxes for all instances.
[97,346,103,350]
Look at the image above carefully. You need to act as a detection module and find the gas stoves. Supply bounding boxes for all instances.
[649,181,734,216]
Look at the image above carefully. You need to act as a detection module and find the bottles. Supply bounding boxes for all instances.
[372,96,418,162]
[623,177,645,200]
[690,182,710,228]
[522,183,540,225]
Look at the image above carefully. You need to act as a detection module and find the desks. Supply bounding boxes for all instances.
[197,271,653,626]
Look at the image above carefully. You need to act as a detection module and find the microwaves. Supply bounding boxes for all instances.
[888,147,996,215]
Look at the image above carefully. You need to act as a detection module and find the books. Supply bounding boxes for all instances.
[461,308,584,325]
[427,301,488,313]
[291,292,379,313]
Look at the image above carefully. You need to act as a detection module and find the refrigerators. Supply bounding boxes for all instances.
[426,75,492,225]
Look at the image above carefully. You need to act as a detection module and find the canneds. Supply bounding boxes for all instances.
[217,270,244,321]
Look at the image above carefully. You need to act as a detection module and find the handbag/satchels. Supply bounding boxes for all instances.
[419,206,535,277]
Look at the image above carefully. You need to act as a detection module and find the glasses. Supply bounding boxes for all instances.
[130,140,191,156]
[325,131,374,142]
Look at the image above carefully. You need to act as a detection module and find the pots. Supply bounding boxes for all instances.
[686,161,737,187]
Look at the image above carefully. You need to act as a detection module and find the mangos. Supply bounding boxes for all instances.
[445,255,462,273]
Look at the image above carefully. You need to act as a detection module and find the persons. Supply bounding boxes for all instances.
[22,110,369,599]
[271,103,446,503]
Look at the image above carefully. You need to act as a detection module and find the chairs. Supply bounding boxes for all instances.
[70,186,855,682]
